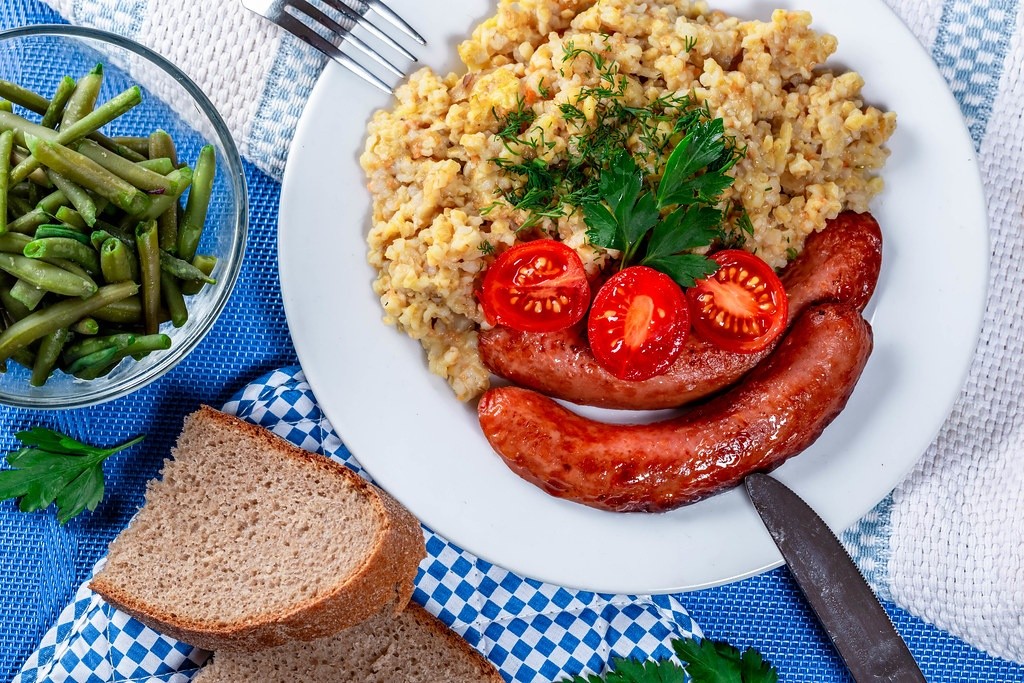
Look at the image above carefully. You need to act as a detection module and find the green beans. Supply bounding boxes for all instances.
[0,63,216,386]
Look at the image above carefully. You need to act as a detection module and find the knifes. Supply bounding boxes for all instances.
[744,472,927,683]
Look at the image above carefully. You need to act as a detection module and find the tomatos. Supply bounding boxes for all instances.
[588,266,687,383]
[484,238,591,332]
[690,249,789,354]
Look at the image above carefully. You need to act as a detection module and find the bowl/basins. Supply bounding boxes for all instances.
[1,23,249,408]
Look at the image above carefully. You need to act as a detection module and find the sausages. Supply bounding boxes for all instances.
[476,208,883,514]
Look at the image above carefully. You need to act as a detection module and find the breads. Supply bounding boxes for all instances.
[90,403,508,683]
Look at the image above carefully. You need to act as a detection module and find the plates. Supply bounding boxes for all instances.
[279,0,989,594]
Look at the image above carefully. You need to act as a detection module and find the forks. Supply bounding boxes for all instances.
[241,1,428,97]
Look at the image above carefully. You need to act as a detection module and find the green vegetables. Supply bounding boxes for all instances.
[550,636,778,683]
[483,36,754,287]
[0,427,145,526]
